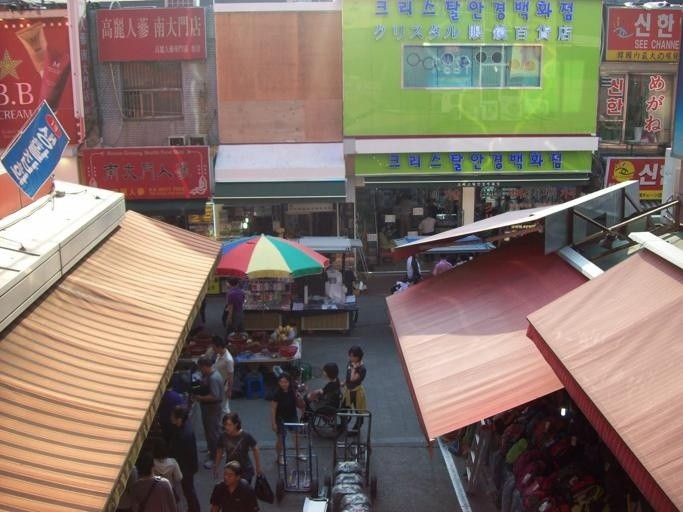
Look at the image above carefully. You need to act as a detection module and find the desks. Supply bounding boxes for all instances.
[241,300,357,335]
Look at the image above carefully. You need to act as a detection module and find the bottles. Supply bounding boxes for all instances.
[238,278,291,309]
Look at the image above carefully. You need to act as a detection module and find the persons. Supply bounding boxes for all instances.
[339,344,367,430]
[166,403,202,512]
[118,452,177,511]
[221,279,232,330]
[224,277,245,333]
[188,357,224,470]
[212,413,262,485]
[209,336,234,416]
[270,371,308,465]
[152,442,190,511]
[297,363,342,424]
[158,381,185,440]
[209,460,260,511]
[309,193,475,299]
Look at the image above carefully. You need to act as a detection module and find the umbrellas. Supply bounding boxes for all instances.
[207,230,330,312]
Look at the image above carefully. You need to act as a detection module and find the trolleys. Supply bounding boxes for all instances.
[275,415,318,498]
[323,407,376,498]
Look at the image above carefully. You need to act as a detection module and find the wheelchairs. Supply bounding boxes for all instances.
[296,387,352,440]
